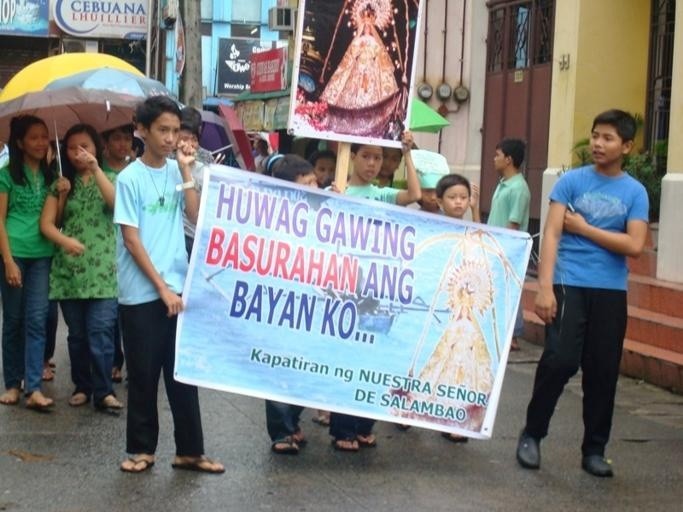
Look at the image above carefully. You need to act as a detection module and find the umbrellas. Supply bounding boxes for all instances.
[0,52,173,179]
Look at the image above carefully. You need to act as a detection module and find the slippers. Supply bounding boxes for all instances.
[270,410,379,455]
[121,450,226,474]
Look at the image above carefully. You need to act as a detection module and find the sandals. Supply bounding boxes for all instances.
[0,364,125,412]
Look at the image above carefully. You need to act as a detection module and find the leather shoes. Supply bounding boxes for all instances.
[581,454,614,478]
[517,429,541,471]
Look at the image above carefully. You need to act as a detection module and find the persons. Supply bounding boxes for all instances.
[488,138,531,350]
[516,109,649,478]
[319,0,400,136]
